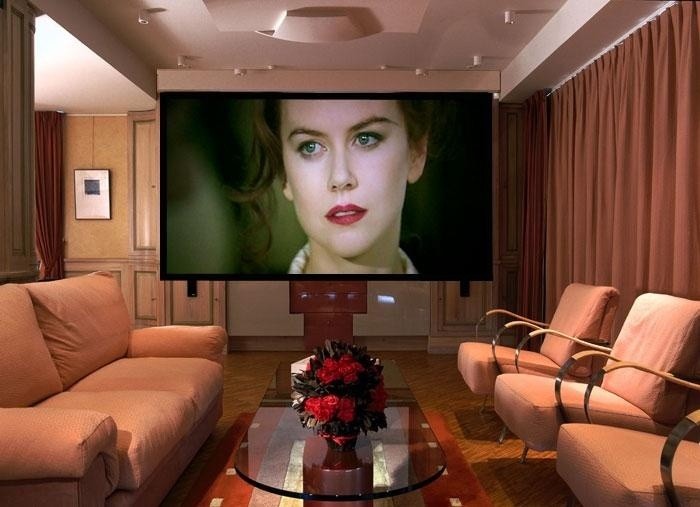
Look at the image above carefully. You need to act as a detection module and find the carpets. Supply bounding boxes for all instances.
[181,406,495,507]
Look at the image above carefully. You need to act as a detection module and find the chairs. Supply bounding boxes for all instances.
[458,280,621,443]
[489,286,698,473]
[558,362,699,506]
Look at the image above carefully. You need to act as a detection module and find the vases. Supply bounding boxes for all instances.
[319,426,359,449]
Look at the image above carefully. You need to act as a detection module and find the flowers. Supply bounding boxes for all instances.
[286,336,389,446]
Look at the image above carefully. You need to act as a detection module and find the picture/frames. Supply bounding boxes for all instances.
[73,168,112,221]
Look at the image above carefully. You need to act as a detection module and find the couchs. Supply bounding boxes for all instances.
[0,265,233,506]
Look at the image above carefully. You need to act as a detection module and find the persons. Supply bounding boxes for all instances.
[221,97,434,276]
